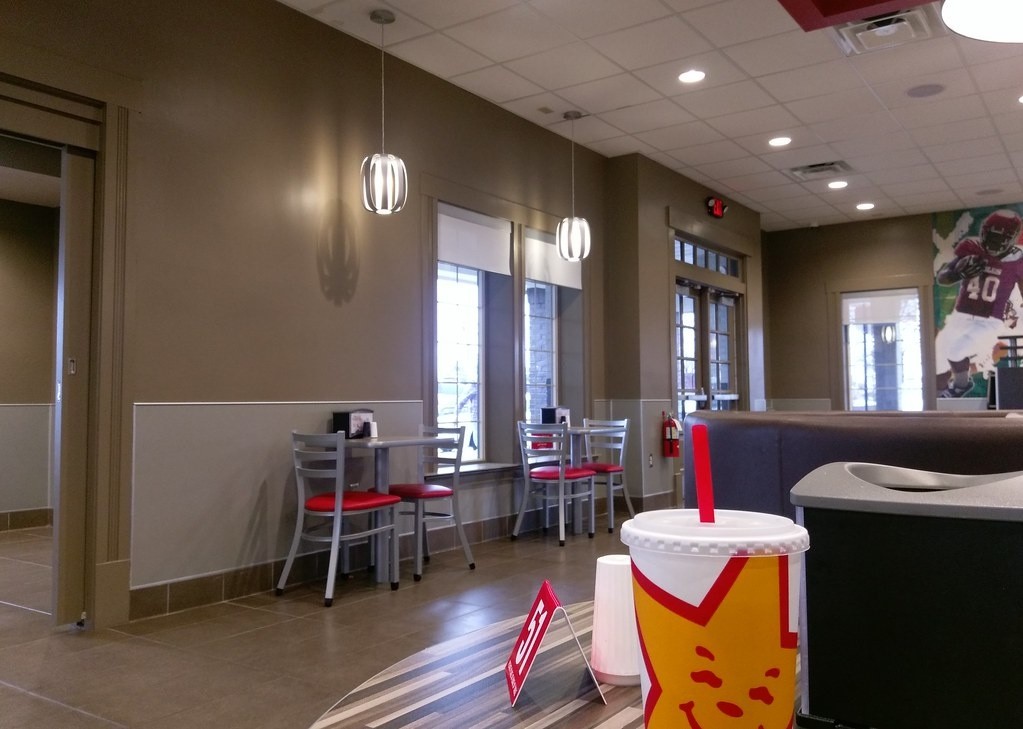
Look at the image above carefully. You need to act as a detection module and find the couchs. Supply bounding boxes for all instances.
[683,411,1023,524]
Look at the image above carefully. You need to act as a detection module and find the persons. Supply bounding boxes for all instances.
[934,209,1023,398]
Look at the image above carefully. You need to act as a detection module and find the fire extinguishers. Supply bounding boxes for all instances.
[662,411,683,457]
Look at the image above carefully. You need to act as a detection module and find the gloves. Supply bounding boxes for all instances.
[956,254,987,278]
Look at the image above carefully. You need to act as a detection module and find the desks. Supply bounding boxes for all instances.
[310,602,802,729]
[305,436,453,587]
[524,426,627,537]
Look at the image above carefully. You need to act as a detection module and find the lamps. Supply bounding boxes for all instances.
[360,9,408,215]
[556,111,592,263]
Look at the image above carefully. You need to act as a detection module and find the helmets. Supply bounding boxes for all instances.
[980,210,1023,257]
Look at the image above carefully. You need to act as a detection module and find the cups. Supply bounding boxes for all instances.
[620,509,810,729]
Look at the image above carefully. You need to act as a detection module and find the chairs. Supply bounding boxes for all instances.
[565,418,634,533]
[274,430,401,608]
[365,425,476,582]
[513,421,597,546]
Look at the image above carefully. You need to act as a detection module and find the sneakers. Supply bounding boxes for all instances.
[938,377,974,397]
